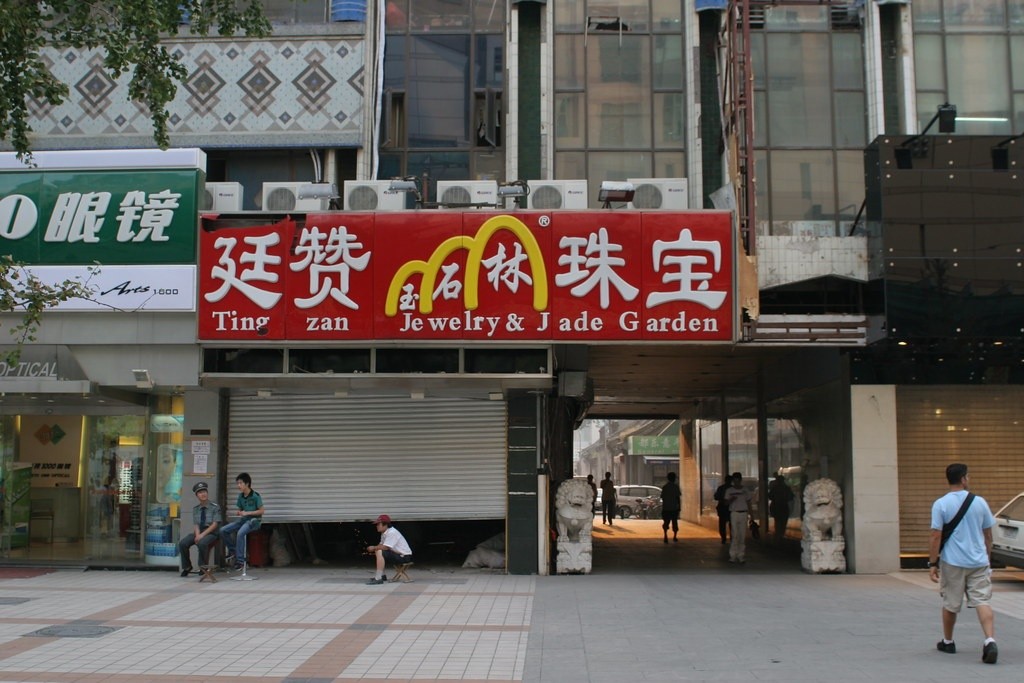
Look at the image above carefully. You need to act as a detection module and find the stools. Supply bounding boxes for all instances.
[199,565,219,582]
[391,562,414,583]
[229,527,259,580]
[197,537,220,573]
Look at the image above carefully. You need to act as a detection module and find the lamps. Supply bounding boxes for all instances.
[335,392,348,399]
[990,134,1024,173]
[296,179,341,210]
[411,393,424,399]
[895,133,922,170]
[498,181,530,208]
[133,370,155,390]
[597,181,635,209]
[257,390,271,398]
[388,175,423,210]
[920,103,957,137]
[489,393,503,400]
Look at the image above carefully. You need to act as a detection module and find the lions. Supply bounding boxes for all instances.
[555,479,594,543]
[801,478,843,541]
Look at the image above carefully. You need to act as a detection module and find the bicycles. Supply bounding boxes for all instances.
[633,495,663,519]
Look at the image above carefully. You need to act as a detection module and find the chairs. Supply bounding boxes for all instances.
[27,497,55,548]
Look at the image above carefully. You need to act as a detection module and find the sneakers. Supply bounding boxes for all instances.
[937,639,955,653]
[982,642,998,663]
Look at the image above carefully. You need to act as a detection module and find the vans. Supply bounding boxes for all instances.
[612,485,663,519]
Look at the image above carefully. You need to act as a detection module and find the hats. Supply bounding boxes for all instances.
[372,515,391,524]
[192,482,208,495]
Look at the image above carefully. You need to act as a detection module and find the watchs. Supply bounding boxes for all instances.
[927,562,937,567]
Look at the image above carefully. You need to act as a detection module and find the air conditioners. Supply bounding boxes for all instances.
[262,181,323,211]
[527,179,588,210]
[436,180,498,210]
[344,180,407,211]
[626,178,688,210]
[205,181,244,212]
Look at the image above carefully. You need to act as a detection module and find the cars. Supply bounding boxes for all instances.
[594,488,603,511]
[988,491,1024,571]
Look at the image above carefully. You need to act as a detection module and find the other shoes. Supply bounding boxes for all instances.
[370,575,387,581]
[673,537,677,541]
[664,537,668,544]
[226,552,235,559]
[366,579,383,585]
[180,566,193,576]
[199,568,209,575]
[236,563,244,570]
[721,537,726,542]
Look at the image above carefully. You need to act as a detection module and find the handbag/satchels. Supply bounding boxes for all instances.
[749,520,760,538]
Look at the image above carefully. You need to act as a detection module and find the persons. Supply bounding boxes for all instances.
[714,475,733,544]
[220,473,264,572]
[587,474,597,517]
[179,482,223,577]
[929,464,999,664]
[725,472,754,564]
[600,472,614,525]
[660,472,681,544]
[366,515,412,585]
[770,472,795,537]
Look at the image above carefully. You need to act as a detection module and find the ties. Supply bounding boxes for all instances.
[200,507,206,531]
